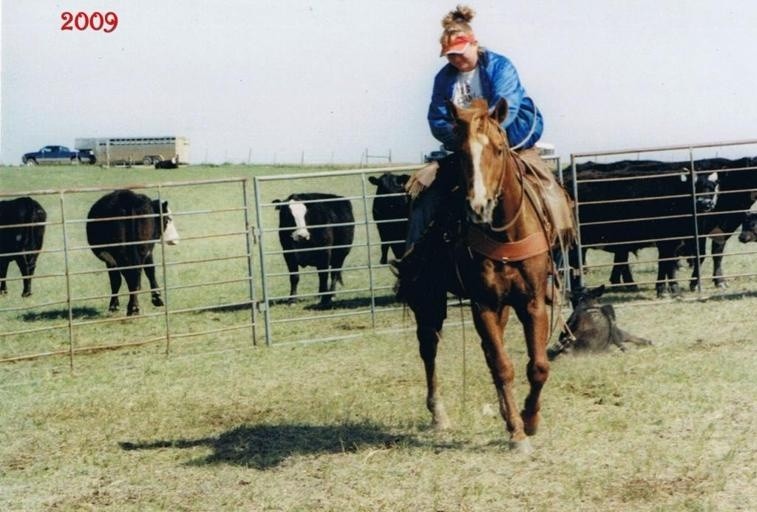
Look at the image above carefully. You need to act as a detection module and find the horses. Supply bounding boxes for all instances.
[387,95,578,463]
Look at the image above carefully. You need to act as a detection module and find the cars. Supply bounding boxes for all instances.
[427,150,449,162]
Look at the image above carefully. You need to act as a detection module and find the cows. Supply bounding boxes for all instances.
[737,211,757,243]
[0,195,48,298]
[553,156,757,294]
[545,283,652,362]
[368,170,413,265]
[85,188,180,317]
[271,191,355,310]
[550,165,720,298]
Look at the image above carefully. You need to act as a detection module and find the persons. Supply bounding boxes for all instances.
[401,3,544,247]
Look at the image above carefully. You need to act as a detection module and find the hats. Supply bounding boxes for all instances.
[438,34,479,59]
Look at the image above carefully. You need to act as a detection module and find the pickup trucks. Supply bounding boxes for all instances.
[21,143,97,166]
